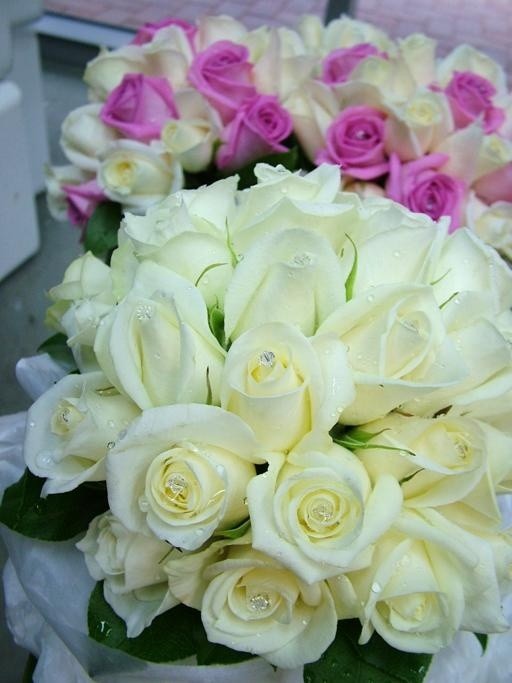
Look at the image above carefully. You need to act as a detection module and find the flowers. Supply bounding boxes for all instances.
[3,161,512,682]
[45,15,511,259]
